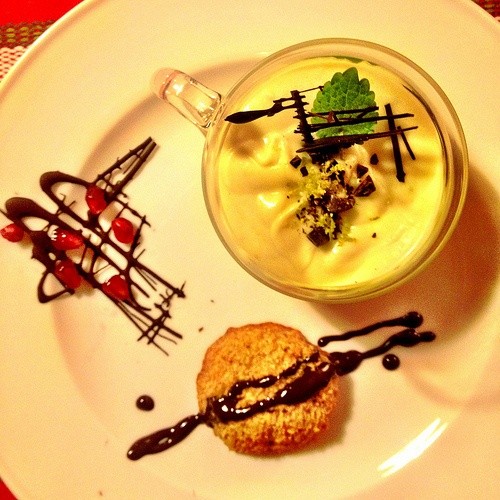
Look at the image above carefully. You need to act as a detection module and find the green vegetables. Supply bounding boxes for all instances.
[309,67,379,139]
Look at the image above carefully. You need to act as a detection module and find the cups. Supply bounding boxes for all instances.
[151,38,469,304]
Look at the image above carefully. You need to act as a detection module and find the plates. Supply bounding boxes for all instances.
[0,0,500,500]
